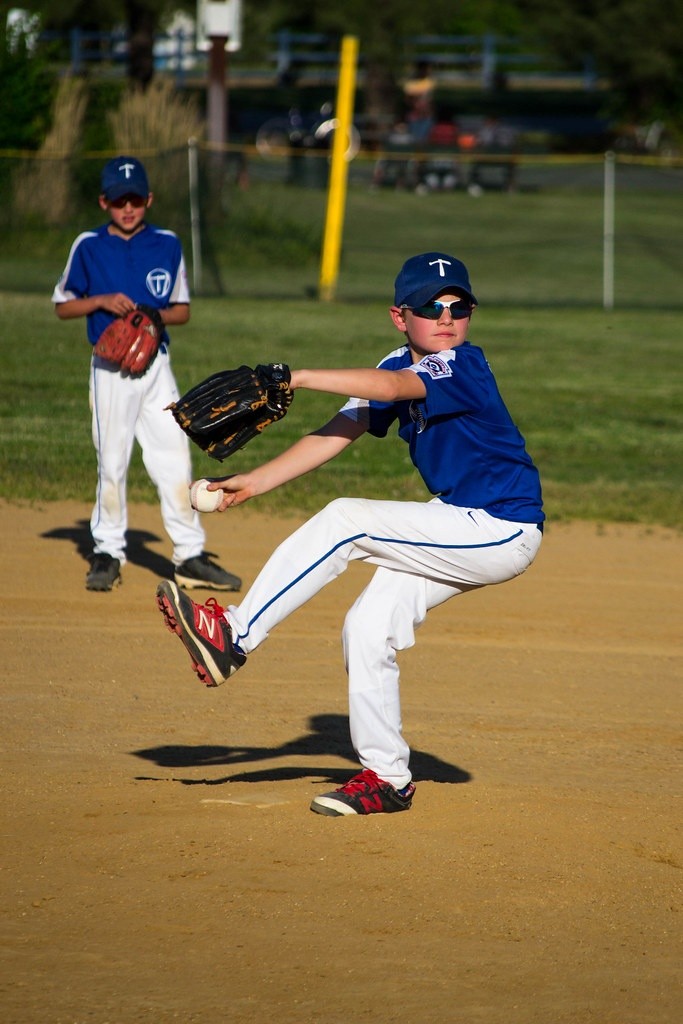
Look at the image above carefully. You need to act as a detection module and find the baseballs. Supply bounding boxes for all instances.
[189,478,225,513]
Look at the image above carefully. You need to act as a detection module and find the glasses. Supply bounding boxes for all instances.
[103,193,147,208]
[399,299,472,320]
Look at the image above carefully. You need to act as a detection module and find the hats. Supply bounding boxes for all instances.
[394,253,478,310]
[100,156,148,200]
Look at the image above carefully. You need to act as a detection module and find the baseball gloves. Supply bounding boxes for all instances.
[161,360,295,463]
[90,302,166,376]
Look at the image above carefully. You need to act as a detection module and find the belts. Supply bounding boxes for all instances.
[537,522,543,534]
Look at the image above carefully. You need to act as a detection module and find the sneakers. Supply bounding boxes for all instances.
[174,552,242,591]
[156,581,247,687]
[311,769,417,817]
[85,553,122,591]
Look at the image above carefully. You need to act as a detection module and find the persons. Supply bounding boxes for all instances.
[155,251,545,818]
[51,157,243,594]
